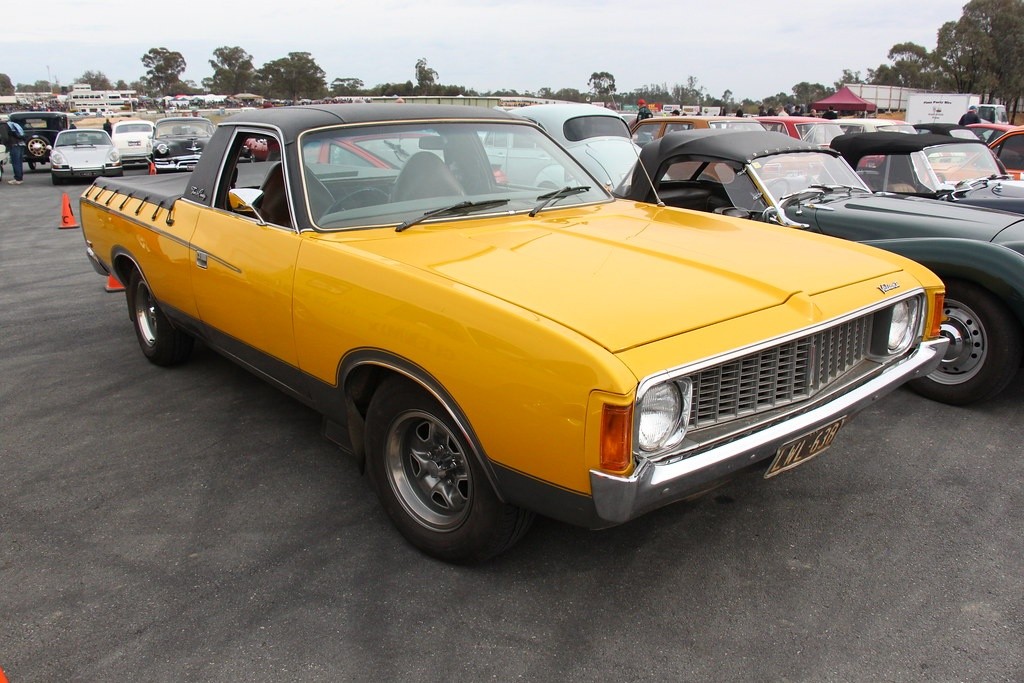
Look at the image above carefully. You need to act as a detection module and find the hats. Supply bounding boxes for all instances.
[969,106,977,110]
[636,99,645,105]
[670,110,680,115]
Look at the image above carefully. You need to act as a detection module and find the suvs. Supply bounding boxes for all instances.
[8,112,77,170]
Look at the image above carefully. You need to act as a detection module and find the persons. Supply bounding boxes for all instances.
[821,105,837,119]
[396,98,405,103]
[69,120,77,137]
[958,106,981,126]
[0,115,26,185]
[791,106,803,138]
[767,108,778,131]
[104,118,112,138]
[668,109,744,134]
[636,99,653,148]
[778,106,789,132]
[804,109,817,141]
[181,109,201,118]
[284,96,372,106]
[0,103,66,114]
[758,104,767,130]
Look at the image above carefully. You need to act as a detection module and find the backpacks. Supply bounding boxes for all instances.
[6,121,26,138]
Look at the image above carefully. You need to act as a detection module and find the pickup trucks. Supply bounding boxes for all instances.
[80,104,950,563]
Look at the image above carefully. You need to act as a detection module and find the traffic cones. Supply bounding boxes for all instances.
[57,193,80,229]
[103,274,126,293]
[150,163,157,175]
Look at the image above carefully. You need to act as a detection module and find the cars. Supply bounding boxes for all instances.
[111,122,155,166]
[242,116,1024,406]
[148,117,216,170]
[0,145,10,181]
[46,129,124,185]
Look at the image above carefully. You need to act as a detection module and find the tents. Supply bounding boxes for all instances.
[811,85,878,119]
[135,92,265,108]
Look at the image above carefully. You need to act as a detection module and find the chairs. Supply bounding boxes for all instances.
[389,151,467,202]
[255,160,345,230]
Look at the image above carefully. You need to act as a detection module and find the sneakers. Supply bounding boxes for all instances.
[8,179,23,185]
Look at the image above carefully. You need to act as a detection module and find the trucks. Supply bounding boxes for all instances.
[905,92,1009,134]
